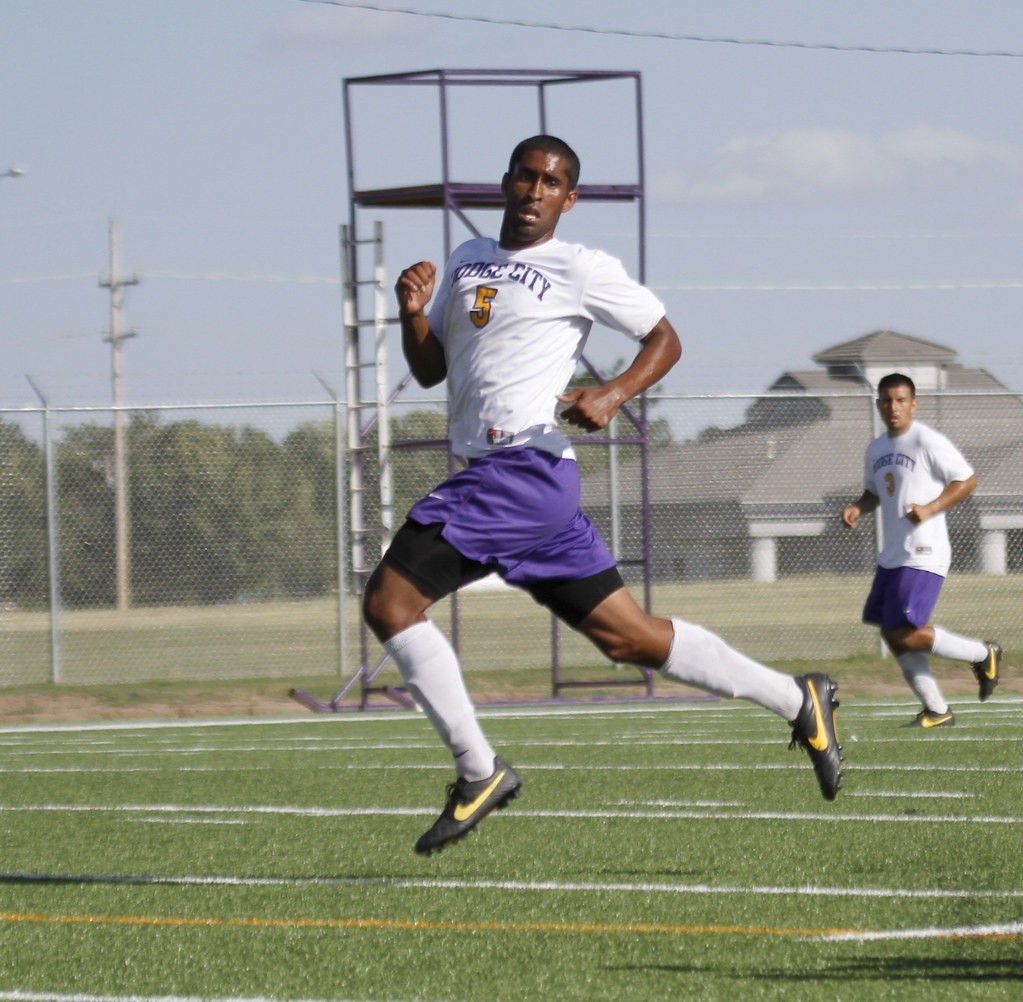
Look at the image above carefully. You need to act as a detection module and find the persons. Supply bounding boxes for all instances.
[361,135,845,858]
[841,373,1003,729]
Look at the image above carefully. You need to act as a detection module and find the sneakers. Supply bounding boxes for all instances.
[971,641,1004,702]
[787,672,845,802]
[899,706,955,728]
[414,756,523,858]
